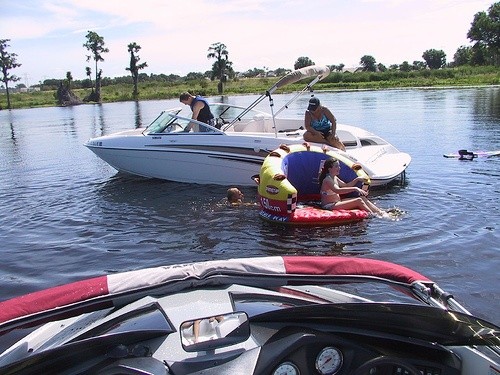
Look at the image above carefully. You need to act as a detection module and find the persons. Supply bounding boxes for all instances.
[226,187,244,204]
[319,157,388,219]
[179,91,215,133]
[303,97,346,153]
[182,316,225,344]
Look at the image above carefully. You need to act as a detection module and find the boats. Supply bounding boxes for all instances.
[83,64,412,188]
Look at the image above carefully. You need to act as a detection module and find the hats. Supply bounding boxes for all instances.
[307,97,320,111]
